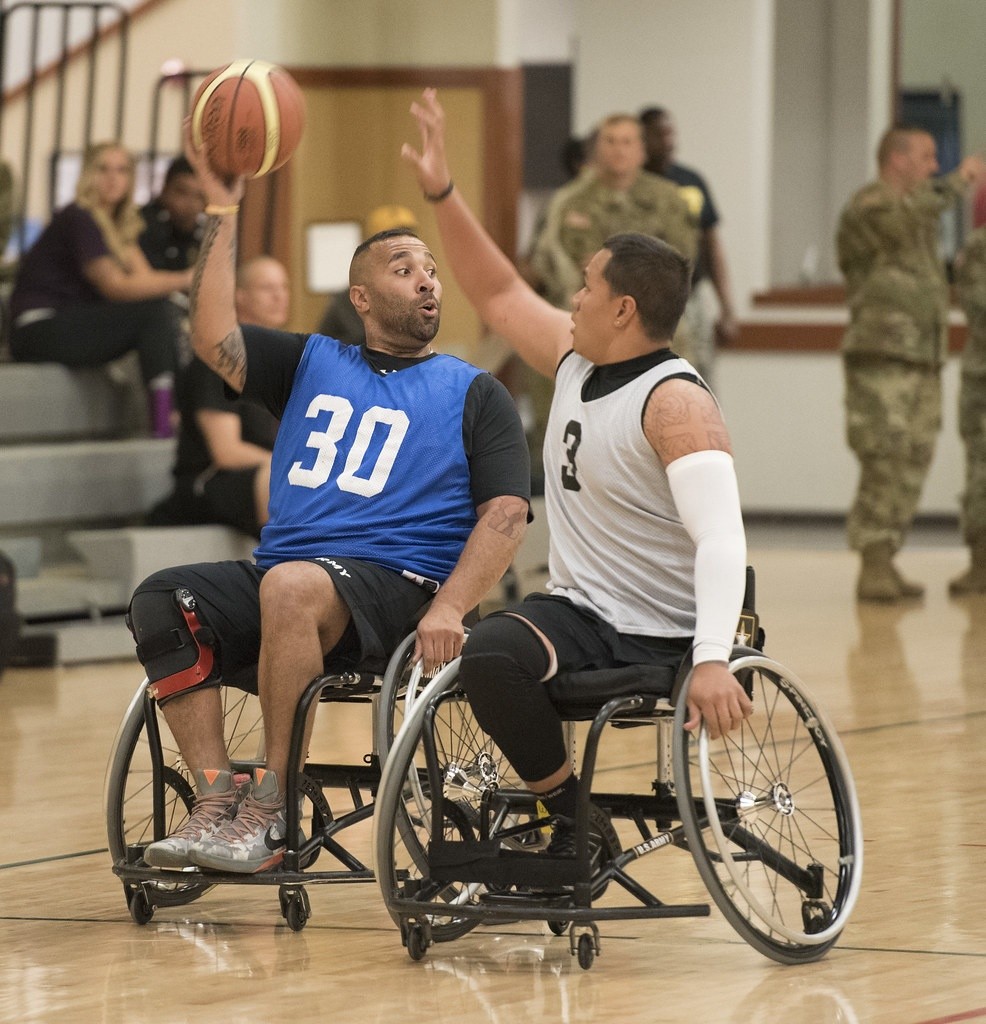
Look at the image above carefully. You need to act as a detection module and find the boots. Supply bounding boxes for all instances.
[858,545,924,602]
[947,546,986,595]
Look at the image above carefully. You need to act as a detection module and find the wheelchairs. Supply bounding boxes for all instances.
[103,625,578,933]
[370,564,865,965]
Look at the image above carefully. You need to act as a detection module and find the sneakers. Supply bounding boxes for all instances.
[143,769,253,867]
[189,768,315,874]
[531,802,614,900]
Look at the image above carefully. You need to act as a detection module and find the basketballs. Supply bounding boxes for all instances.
[189,58,308,181]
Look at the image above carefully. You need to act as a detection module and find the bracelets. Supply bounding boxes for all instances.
[423,178,455,204]
[205,204,240,215]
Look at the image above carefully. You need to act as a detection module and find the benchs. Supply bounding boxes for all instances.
[0,354,260,667]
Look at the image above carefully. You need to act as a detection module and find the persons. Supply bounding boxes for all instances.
[524,104,740,373]
[835,124,986,603]
[397,83,752,889]
[127,139,534,873]
[8,143,306,544]
